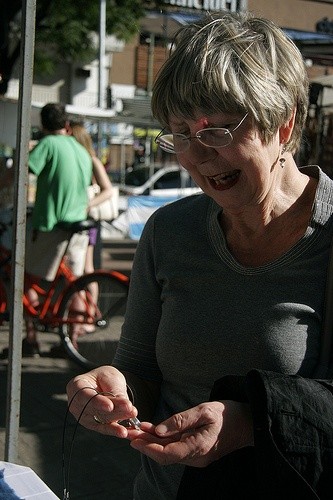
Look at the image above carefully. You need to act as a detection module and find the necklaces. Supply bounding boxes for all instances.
[61,387,141,500]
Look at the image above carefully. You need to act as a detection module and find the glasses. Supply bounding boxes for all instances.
[156,112,254,155]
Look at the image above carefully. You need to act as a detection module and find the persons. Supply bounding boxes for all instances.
[3,102,93,356]
[65,126,114,335]
[66,11,333,500]
[126,143,150,196]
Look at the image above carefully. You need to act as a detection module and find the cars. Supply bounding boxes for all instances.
[112,161,203,196]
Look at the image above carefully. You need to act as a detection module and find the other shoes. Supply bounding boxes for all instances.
[69,324,97,349]
[22,337,39,358]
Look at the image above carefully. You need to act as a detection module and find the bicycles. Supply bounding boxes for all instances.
[0,212,130,369]
